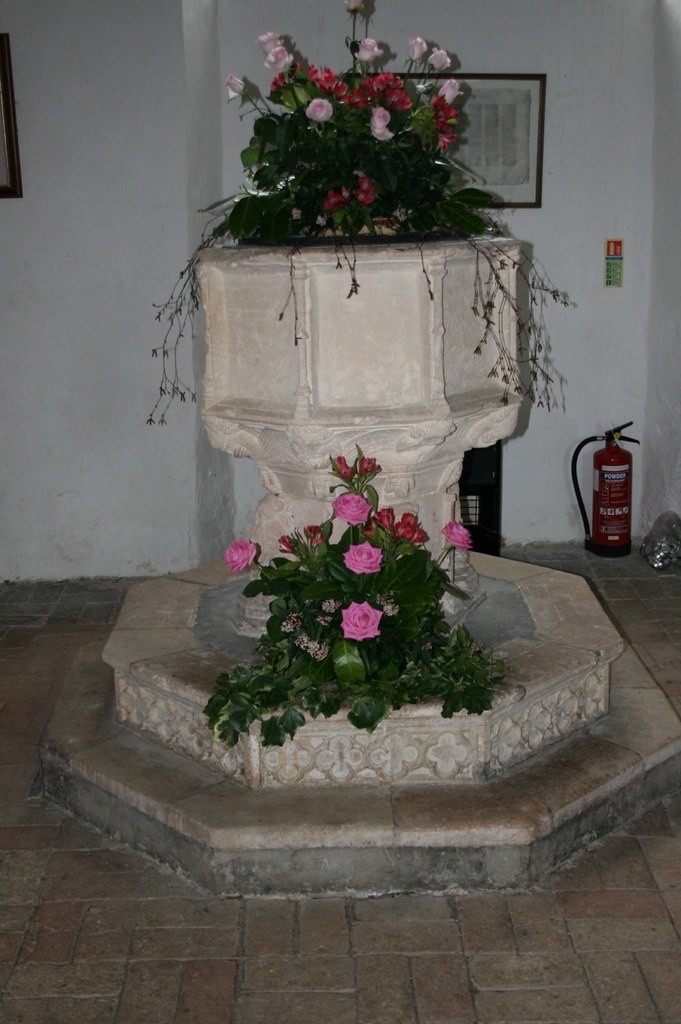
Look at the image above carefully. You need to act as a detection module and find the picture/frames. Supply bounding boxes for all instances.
[0,33,23,199]
[340,72,547,209]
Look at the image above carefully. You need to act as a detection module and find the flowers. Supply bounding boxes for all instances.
[196,443,511,749]
[218,1,500,245]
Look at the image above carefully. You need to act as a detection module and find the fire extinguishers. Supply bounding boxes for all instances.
[570,420,642,558]
